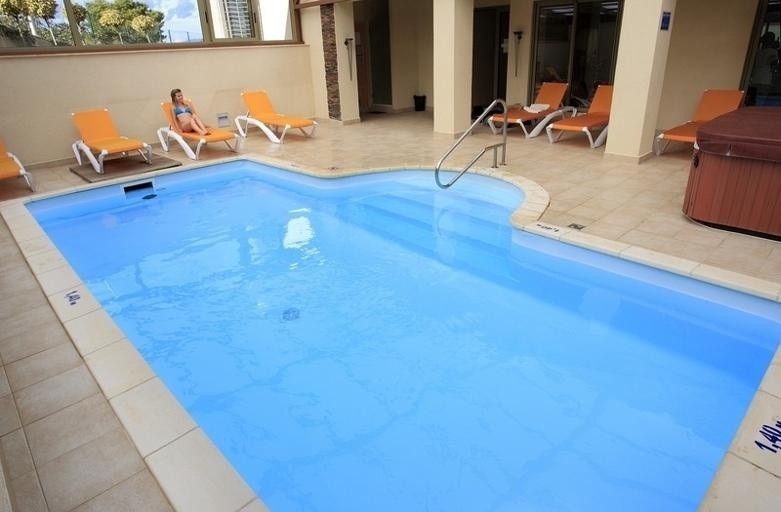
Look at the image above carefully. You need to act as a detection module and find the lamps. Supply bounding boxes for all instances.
[513,31,522,76]
[345,37,353,81]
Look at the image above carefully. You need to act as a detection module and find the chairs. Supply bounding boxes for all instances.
[545,83,614,149]
[69,107,152,173]
[0,136,34,193]
[235,91,317,144]
[655,87,746,156]
[488,82,578,138]
[159,99,241,161]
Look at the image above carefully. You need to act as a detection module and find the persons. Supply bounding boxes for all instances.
[171,89,209,136]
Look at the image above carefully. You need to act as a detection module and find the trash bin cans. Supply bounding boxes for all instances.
[413,95,426,111]
